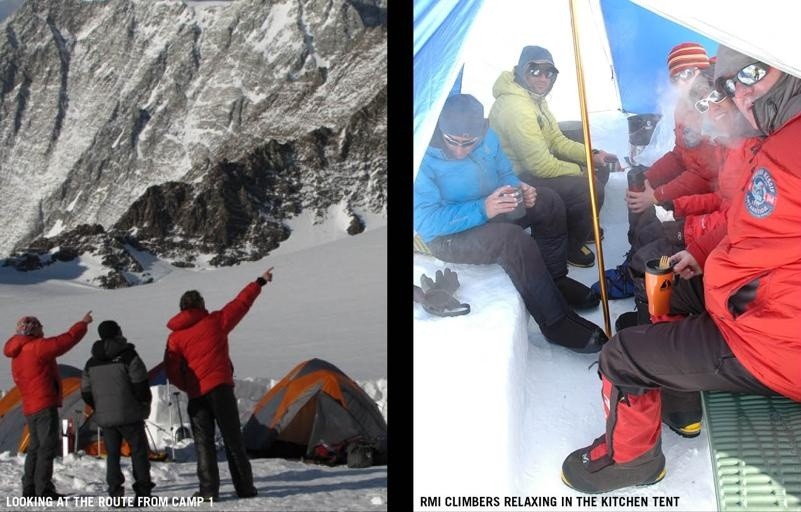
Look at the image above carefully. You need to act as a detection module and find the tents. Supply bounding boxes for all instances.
[242,357,387,467]
[143,360,222,453]
[0,364,131,457]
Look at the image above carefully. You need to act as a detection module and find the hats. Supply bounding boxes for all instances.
[667,42,711,78]
[438,94,484,137]
[16,315,38,335]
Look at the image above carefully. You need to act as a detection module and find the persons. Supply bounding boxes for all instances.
[165,266,274,506]
[414,94,610,354]
[561,42,801,495]
[488,45,611,267]
[589,43,717,299]
[80,321,158,508]
[615,67,765,332]
[3,310,93,502]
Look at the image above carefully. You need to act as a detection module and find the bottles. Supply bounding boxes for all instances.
[604,154,621,172]
[626,167,647,216]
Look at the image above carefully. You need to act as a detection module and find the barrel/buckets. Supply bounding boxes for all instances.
[627,112,664,164]
[557,120,585,146]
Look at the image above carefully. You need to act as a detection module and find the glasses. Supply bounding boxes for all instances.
[694,90,727,113]
[671,68,698,84]
[719,62,768,97]
[528,67,554,79]
[443,134,479,147]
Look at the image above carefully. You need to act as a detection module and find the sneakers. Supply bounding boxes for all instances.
[663,411,703,438]
[543,313,608,353]
[568,245,595,267]
[592,260,632,299]
[560,443,666,495]
[587,227,604,243]
[559,277,600,309]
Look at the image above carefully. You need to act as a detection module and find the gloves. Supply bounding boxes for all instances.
[413,268,471,318]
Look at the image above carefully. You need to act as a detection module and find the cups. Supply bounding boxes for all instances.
[498,181,527,220]
[644,256,677,318]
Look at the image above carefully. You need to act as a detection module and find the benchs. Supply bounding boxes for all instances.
[701,387,800,512]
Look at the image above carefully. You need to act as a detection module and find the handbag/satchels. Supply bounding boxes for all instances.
[312,442,373,468]
